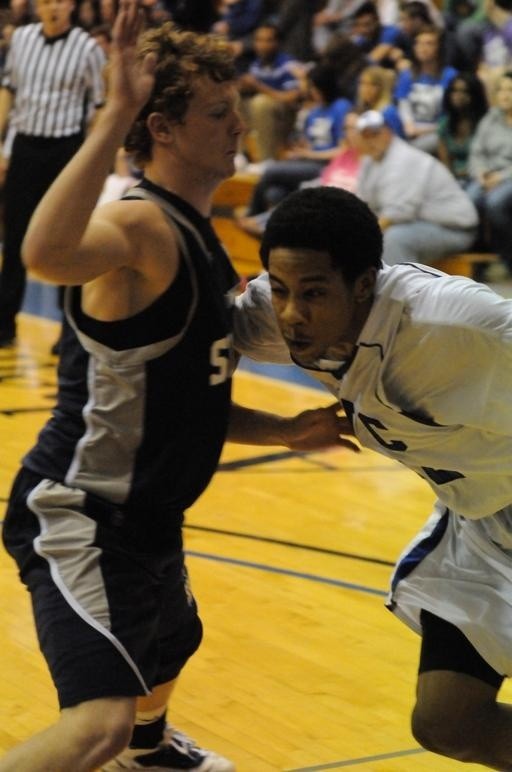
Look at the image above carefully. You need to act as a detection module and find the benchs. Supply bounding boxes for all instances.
[211,217,499,282]
[211,176,266,208]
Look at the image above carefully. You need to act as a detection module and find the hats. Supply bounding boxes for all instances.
[355,110,383,132]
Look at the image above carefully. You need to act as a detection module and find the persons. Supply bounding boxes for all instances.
[0,3,359,772]
[0,1,108,349]
[1,0,512,267]
[231,186,512,772]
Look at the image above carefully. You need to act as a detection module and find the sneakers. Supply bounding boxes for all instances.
[103,727,232,771]
